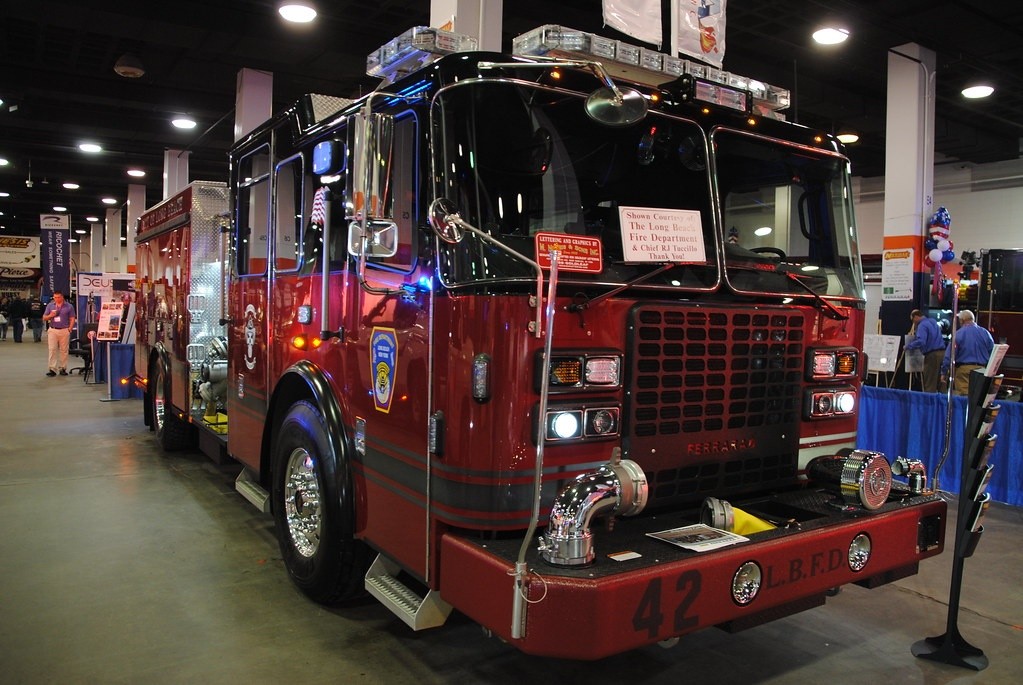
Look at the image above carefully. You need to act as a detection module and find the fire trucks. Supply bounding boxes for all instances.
[121,21,961,661]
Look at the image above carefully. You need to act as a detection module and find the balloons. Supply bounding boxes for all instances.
[925,206,955,269]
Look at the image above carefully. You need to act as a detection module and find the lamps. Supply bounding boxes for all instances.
[113,54,145,78]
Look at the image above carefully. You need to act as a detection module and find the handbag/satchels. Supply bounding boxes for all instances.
[0,314,7,323]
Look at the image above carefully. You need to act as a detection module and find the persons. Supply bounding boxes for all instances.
[0,289,54,343]
[903,309,945,393]
[43,291,74,376]
[941,309,994,394]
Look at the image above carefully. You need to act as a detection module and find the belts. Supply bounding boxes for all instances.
[51,326,62,329]
[959,363,986,368]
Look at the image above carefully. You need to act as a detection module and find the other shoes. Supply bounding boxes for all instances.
[60,369,68,375]
[46,371,56,376]
[2,338,6,340]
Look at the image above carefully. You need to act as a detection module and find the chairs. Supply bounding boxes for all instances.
[69,323,98,374]
